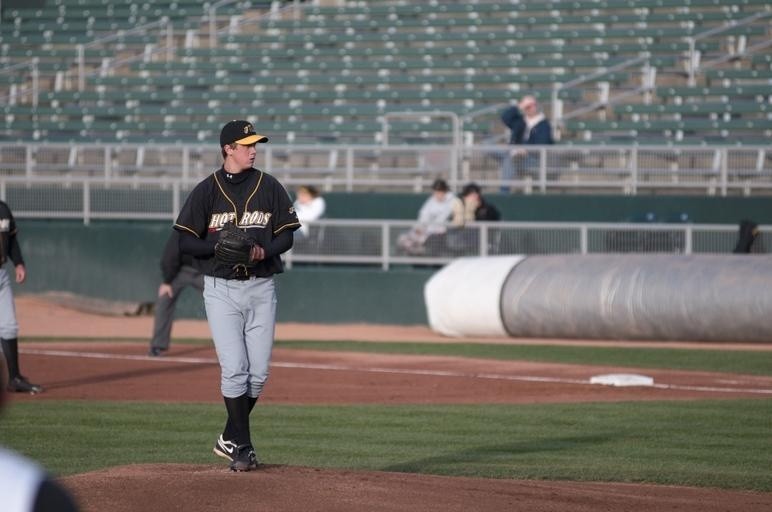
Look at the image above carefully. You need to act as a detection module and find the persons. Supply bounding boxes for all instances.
[1,448,74,511]
[292,185,327,270]
[401,179,457,264]
[157,122,304,472]
[147,225,208,359]
[448,182,501,256]
[497,97,563,194]
[0,199,48,394]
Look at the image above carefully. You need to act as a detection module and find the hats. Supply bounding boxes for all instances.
[220,119,269,148]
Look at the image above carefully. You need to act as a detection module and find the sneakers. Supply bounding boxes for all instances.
[8,375,42,393]
[212,433,235,461]
[231,443,257,471]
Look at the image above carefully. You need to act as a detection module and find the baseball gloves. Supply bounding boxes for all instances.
[215,222,256,266]
